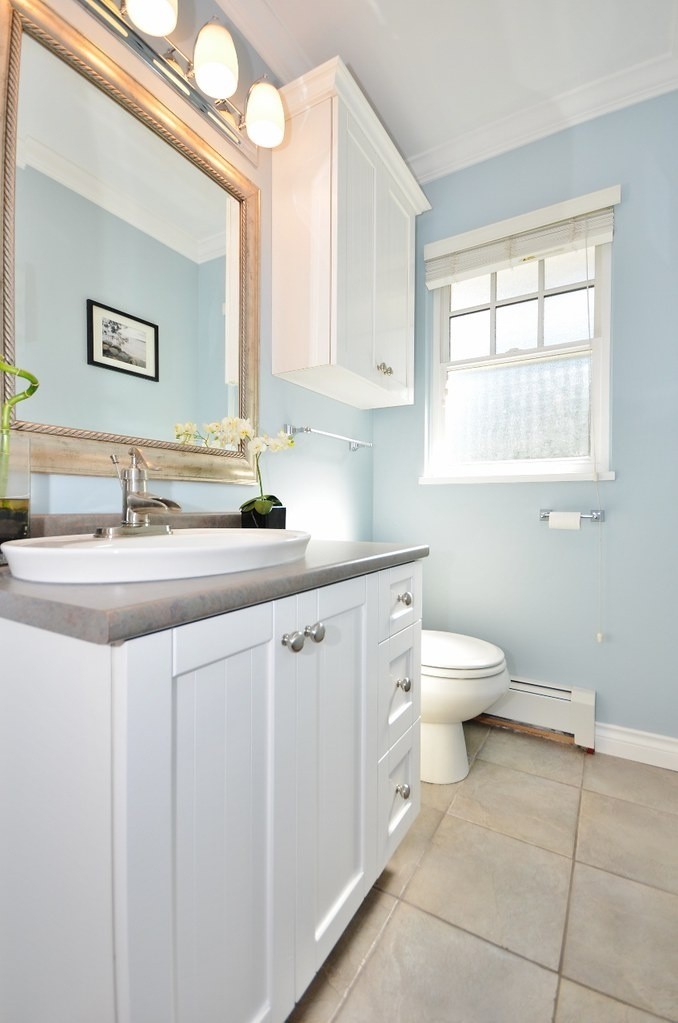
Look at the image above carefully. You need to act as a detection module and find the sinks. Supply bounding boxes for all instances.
[0,527,312,584]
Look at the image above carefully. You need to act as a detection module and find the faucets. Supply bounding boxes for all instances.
[92,446,182,538]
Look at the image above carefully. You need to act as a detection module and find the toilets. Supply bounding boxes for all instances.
[419,629,512,785]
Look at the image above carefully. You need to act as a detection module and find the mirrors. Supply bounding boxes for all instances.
[0,0,263,487]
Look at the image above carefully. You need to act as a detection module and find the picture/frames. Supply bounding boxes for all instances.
[86,299,159,381]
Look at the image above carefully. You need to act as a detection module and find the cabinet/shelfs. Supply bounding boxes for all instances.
[270,54,431,411]
[0,560,422,1023]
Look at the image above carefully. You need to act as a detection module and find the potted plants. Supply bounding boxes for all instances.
[0,361,39,542]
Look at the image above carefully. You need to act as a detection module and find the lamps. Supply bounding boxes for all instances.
[238,73,285,147]
[188,15,239,100]
[118,0,178,37]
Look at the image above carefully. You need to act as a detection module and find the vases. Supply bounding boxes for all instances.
[241,509,286,529]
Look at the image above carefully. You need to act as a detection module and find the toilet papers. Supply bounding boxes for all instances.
[548,511,583,530]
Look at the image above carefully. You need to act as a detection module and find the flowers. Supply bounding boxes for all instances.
[219,416,295,514]
[174,420,220,447]
